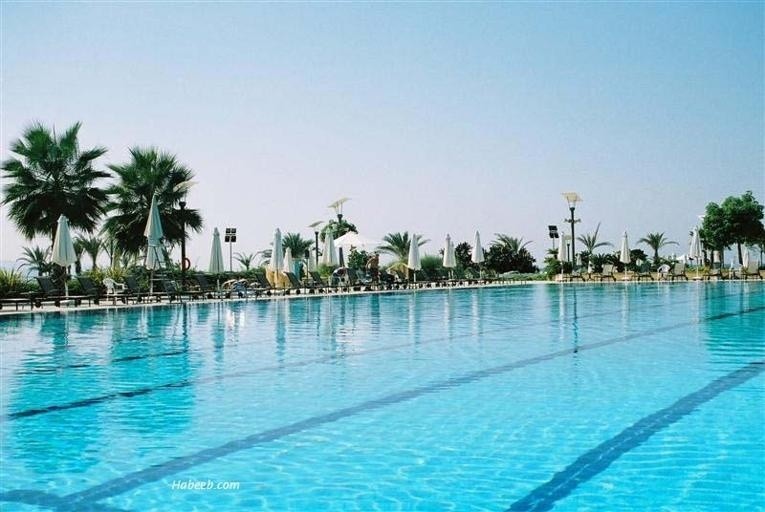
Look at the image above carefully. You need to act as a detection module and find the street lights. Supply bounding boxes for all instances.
[549,225,558,250]
[175,180,198,290]
[331,199,349,266]
[561,192,579,266]
[311,220,322,268]
[225,228,236,272]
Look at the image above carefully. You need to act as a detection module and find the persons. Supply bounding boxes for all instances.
[366,253,380,282]
[226,283,236,290]
[587,264,596,275]
[330,273,339,286]
[337,277,347,286]
[236,282,248,296]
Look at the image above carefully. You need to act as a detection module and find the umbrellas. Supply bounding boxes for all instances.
[743,246,751,269]
[147,244,164,293]
[283,247,292,273]
[620,233,635,274]
[471,232,486,280]
[444,235,460,279]
[207,230,227,291]
[407,235,422,282]
[145,197,161,246]
[321,230,338,286]
[268,230,284,289]
[250,282,263,289]
[575,252,582,273]
[689,228,702,276]
[221,278,236,288]
[50,216,78,297]
[238,277,249,284]
[558,233,569,276]
